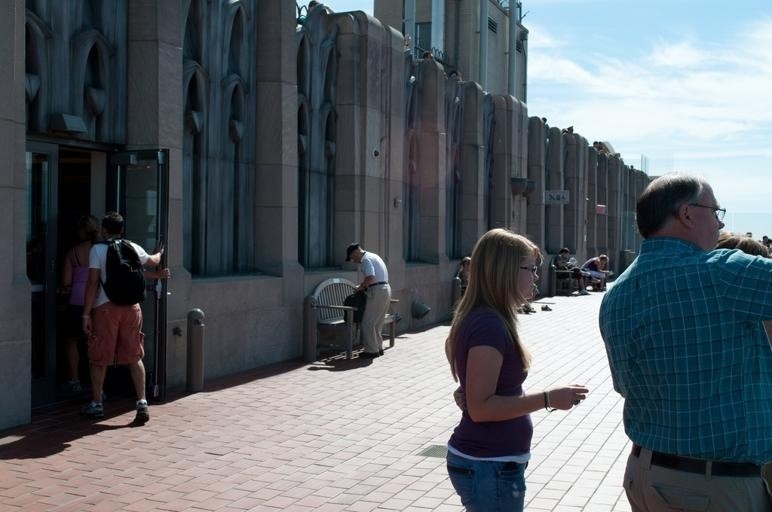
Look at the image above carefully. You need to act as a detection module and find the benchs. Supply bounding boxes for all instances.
[550,256,590,294]
[302,278,400,363]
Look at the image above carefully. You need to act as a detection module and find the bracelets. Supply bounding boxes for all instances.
[79,315,92,320]
[543,388,556,413]
[360,282,369,289]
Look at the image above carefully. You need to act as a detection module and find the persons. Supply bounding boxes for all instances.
[715,228,771,258]
[443,223,589,509]
[597,172,771,511]
[554,246,615,296]
[60,208,171,428]
[456,255,471,297]
[344,241,392,359]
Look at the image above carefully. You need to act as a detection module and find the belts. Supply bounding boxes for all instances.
[631,443,761,478]
[368,281,387,287]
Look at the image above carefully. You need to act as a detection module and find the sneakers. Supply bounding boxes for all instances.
[81,403,104,418]
[134,405,149,425]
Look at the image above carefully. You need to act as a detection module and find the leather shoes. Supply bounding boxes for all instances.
[359,352,379,357]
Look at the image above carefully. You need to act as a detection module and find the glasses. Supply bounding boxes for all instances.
[520,266,537,272]
[688,202,726,220]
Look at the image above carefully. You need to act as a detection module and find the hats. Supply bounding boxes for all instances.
[346,244,360,261]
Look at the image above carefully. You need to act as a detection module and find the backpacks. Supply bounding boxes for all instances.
[343,291,366,322]
[92,237,145,306]
